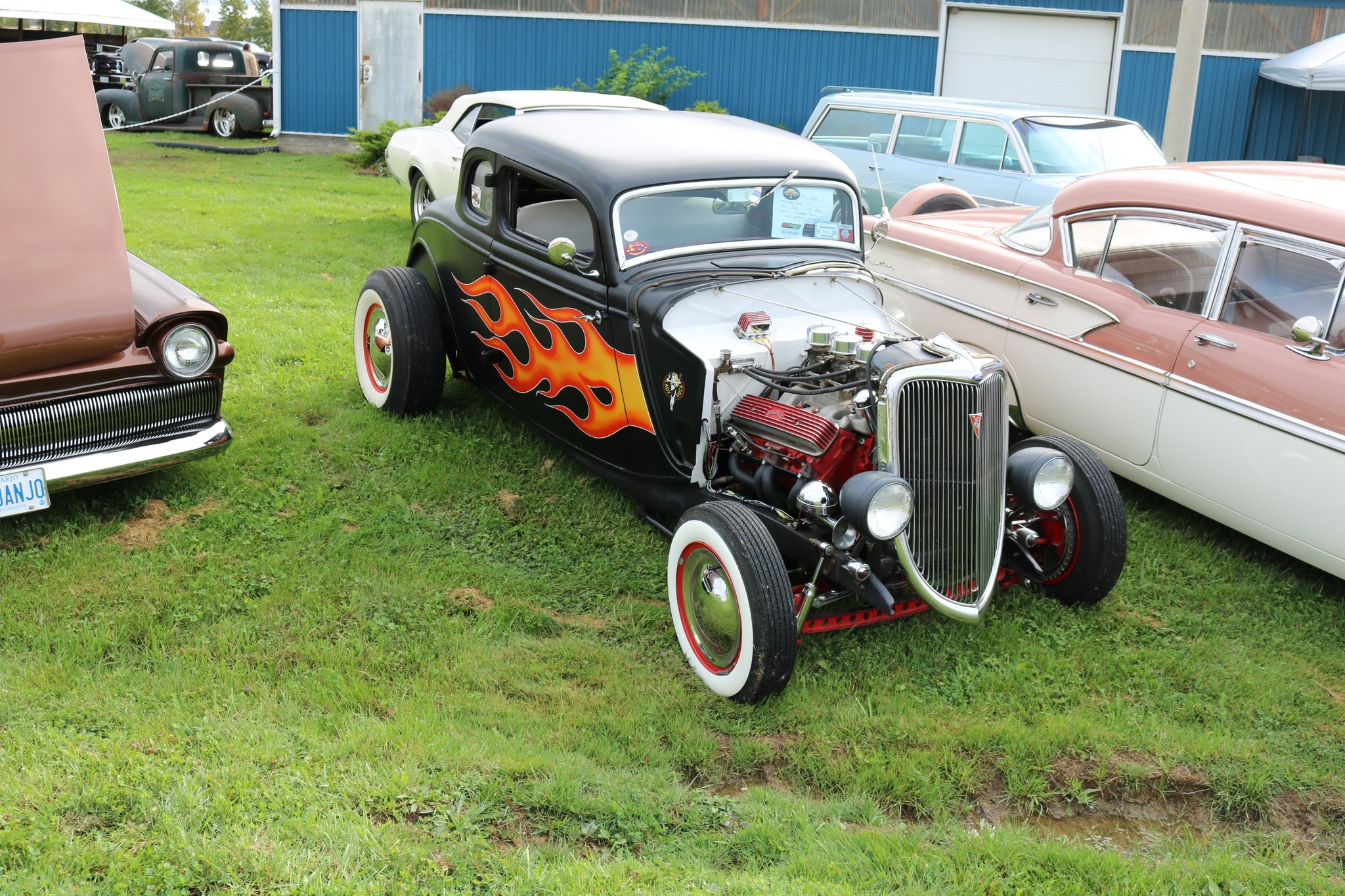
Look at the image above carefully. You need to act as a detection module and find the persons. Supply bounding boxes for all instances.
[241,44,259,76]
[267,59,272,86]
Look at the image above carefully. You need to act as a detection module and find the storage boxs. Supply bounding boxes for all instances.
[868,133,942,157]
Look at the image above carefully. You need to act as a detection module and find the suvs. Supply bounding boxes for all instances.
[862,162,1344,585]
[800,86,1180,214]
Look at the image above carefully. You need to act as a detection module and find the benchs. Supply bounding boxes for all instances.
[515,196,751,260]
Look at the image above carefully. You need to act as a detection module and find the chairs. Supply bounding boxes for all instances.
[964,155,1011,169]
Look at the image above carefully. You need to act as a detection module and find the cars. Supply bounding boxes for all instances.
[351,110,1125,703]
[385,91,672,227]
[89,38,273,92]
[0,35,235,517]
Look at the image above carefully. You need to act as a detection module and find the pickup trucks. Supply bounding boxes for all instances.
[95,42,273,139]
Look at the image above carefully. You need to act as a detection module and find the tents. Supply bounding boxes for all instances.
[1243,31,1345,165]
[0,0,175,46]
